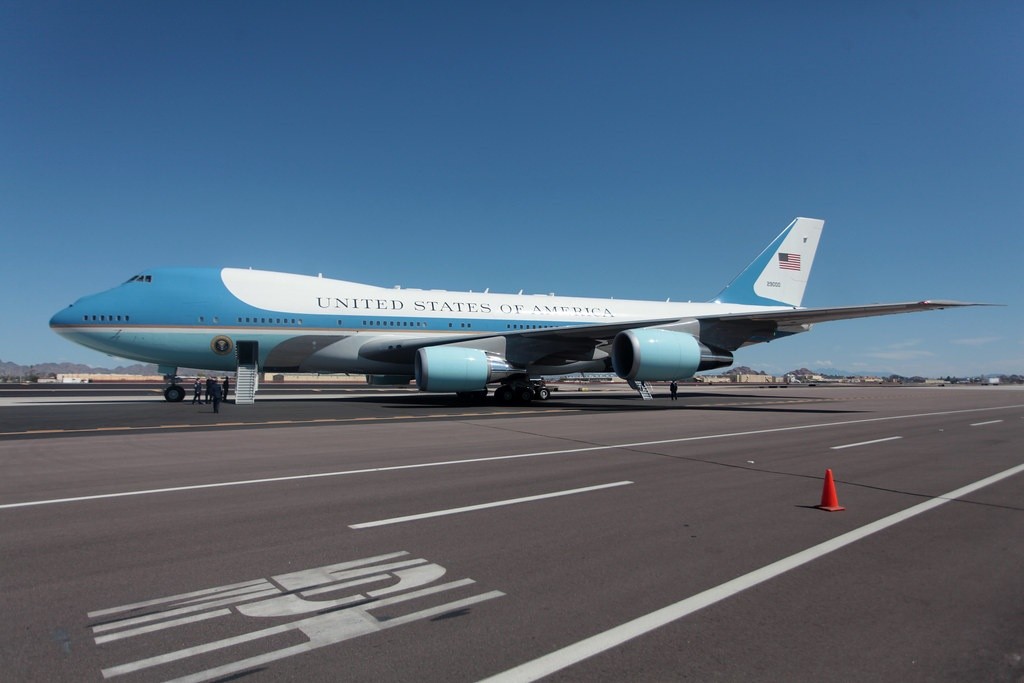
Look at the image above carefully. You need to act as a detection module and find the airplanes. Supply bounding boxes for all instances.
[46,217,1010,406]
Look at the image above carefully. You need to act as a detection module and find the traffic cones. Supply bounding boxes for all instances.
[813,468,846,512]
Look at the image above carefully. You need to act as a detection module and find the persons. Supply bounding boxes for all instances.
[192,375,229,414]
[670,379,678,401]
[641,380,647,391]
